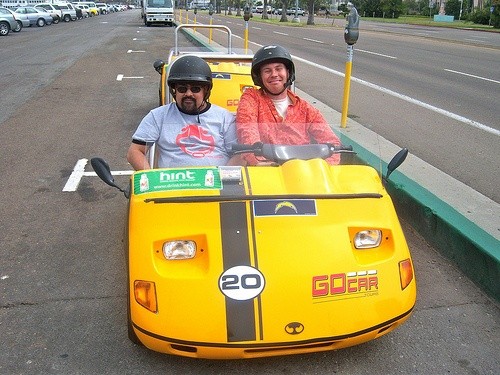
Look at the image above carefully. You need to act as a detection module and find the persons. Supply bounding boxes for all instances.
[126,56,247,171]
[236,43,341,167]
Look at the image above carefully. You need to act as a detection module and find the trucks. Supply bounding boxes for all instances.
[141,0,174,28]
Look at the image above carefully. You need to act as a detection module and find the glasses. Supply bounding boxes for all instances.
[175,85,205,93]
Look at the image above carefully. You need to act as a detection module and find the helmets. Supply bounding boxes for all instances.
[167,56,213,85]
[251,45,295,85]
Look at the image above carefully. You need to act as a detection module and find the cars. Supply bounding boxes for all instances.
[251,4,306,17]
[13,2,128,27]
[6,8,31,32]
[89,24,419,362]
[0,7,18,36]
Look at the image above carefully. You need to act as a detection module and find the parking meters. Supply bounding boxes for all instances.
[185,7,191,27]
[340,6,361,128]
[242,2,254,55]
[194,8,199,33]
[178,6,183,23]
[208,4,214,43]
